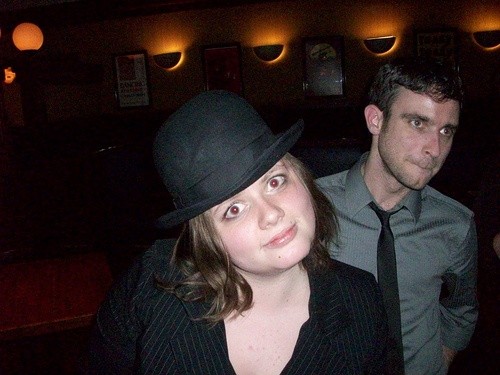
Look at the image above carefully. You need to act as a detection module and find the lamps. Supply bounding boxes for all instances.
[3,67,16,84]
[471,29,500,51]
[364,35,396,55]
[151,52,181,70]
[12,23,44,58]
[255,43,283,63]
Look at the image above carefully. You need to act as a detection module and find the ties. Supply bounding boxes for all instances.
[367,203,404,375]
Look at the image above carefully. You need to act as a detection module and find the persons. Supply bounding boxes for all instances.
[73,90,393,374]
[314,47,478,374]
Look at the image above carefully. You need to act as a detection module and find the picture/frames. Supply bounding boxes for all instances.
[301,36,346,98]
[113,50,149,108]
[199,43,244,98]
[414,31,455,63]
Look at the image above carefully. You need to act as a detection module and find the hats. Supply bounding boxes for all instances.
[150,88,304,231]
